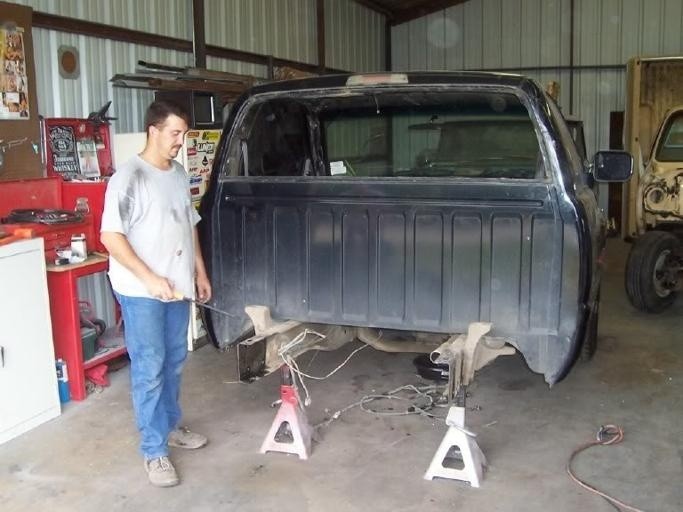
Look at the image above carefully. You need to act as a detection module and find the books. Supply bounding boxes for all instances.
[76,140,101,178]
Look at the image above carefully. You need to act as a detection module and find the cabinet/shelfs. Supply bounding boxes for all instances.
[0,238,60,442]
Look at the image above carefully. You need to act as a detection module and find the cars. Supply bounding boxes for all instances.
[196,72,634,391]
[625,105,683,315]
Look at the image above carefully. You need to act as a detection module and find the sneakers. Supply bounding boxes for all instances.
[167,424,207,450]
[143,453,179,488]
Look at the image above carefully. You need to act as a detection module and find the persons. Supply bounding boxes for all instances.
[100,101,212,485]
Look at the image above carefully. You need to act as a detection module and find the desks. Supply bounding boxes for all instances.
[46,250,127,402]
